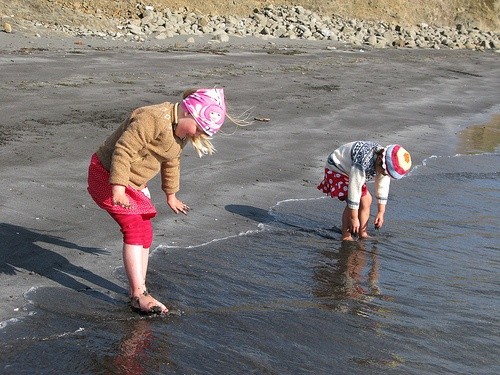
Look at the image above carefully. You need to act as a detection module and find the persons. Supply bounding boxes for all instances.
[101,312,183,375]
[85,86,256,316]
[311,227,405,369]
[316,140,413,241]
